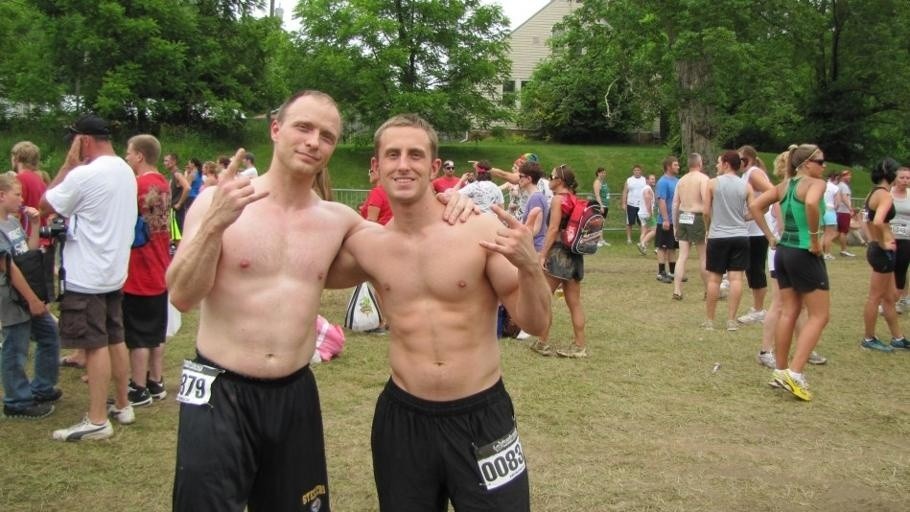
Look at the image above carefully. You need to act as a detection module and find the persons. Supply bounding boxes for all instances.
[511,161,549,339]
[461,158,505,217]
[469,152,549,191]
[323,113,552,511]
[0,172,63,419]
[162,153,191,248]
[529,164,588,359]
[737,146,774,326]
[9,140,56,302]
[622,165,646,245]
[358,166,393,227]
[125,133,167,408]
[879,167,910,314]
[699,151,754,332]
[749,143,830,401]
[165,90,481,511]
[199,161,218,193]
[38,117,139,443]
[592,167,612,248]
[835,167,859,259]
[216,157,230,172]
[862,159,910,351]
[431,157,466,196]
[671,152,726,300]
[654,156,687,283]
[184,157,202,203]
[757,152,827,369]
[638,174,656,255]
[238,152,258,178]
[822,169,843,260]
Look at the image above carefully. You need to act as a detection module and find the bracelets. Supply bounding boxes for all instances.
[808,231,821,237]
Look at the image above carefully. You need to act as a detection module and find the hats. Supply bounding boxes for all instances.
[63,114,113,137]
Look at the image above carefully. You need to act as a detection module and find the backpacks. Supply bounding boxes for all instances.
[551,192,605,256]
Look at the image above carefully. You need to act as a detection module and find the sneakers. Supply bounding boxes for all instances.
[823,253,837,260]
[600,239,611,246]
[107,401,136,426]
[3,402,56,420]
[668,271,688,281]
[839,250,855,257]
[51,411,114,442]
[529,338,551,356]
[656,272,672,284]
[756,349,776,369]
[32,385,63,402]
[556,341,588,358]
[671,293,684,302]
[636,242,648,255]
[889,335,910,350]
[767,369,785,388]
[726,320,739,330]
[146,370,168,400]
[807,350,827,365]
[860,335,895,353]
[106,378,153,409]
[774,369,813,401]
[699,319,714,331]
[737,307,767,323]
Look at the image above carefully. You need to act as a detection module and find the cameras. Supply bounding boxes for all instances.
[39,216,66,243]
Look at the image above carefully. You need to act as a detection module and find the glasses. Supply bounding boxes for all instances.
[446,166,455,170]
[549,174,560,181]
[810,159,825,165]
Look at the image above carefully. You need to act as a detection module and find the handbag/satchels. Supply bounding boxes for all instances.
[7,249,50,312]
